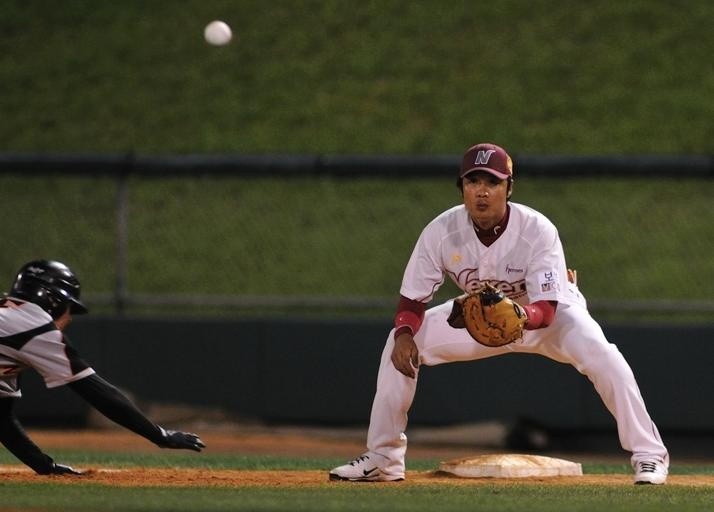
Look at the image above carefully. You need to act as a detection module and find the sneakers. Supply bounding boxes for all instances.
[632,458,671,484]
[329,450,407,482]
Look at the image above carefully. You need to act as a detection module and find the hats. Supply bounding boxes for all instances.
[460,143,514,182]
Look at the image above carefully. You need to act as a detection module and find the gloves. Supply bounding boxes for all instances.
[50,462,83,475]
[567,268,577,286]
[155,424,206,452]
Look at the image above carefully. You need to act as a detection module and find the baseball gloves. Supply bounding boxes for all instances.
[447,284,525,346]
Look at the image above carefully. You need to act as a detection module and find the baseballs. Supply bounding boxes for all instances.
[205,21,231,44]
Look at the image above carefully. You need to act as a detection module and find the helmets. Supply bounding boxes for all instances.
[9,258,88,321]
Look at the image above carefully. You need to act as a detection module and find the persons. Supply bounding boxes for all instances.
[328,141,672,487]
[0,255,209,478]
